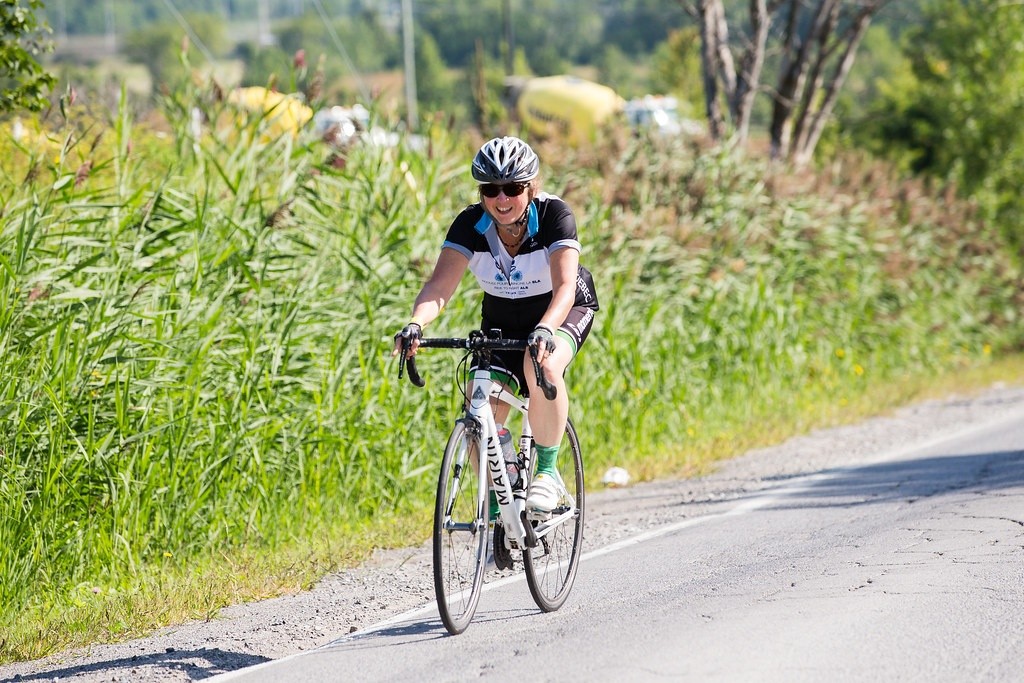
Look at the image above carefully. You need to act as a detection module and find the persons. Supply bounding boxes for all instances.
[391,136,599,574]
[495,74,629,149]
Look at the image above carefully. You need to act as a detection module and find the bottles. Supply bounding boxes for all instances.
[497,420,520,489]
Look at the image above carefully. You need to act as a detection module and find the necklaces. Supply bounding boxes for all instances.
[493,213,529,248]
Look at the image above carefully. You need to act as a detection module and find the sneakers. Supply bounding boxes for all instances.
[478,521,499,571]
[524,469,565,510]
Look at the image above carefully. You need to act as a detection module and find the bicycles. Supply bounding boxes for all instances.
[397,324,587,633]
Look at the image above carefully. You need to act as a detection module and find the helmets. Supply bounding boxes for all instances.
[471,136,540,185]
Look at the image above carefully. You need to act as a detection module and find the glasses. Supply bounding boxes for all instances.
[481,183,530,198]
